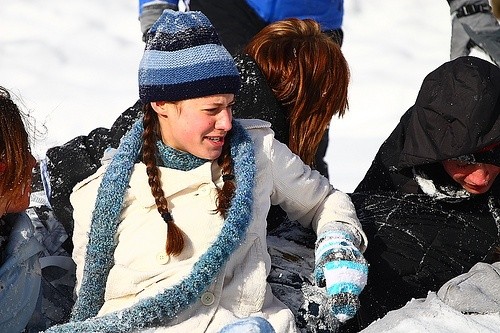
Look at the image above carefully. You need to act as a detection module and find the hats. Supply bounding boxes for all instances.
[139,7,241,102]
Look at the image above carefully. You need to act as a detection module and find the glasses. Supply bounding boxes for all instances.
[0,142,32,164]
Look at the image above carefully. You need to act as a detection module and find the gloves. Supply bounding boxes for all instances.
[315,220,368,322]
[449,0,500,68]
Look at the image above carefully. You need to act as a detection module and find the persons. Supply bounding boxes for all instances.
[138,0,500,180]
[0,9,500,333]
